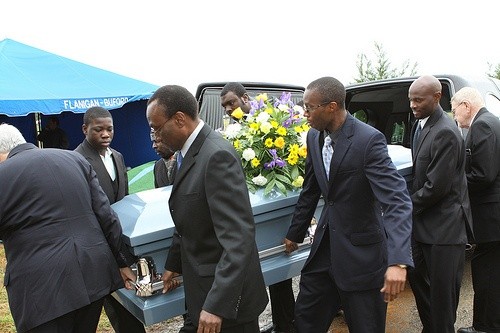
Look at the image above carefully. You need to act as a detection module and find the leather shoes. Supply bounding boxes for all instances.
[457,326,488,333]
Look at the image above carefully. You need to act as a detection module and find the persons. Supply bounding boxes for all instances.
[147,128,181,188]
[144,87,269,333]
[409,74,477,333]
[451,86,500,332]
[282,76,415,333]
[72,106,129,207]
[220,83,254,122]
[0,124,138,333]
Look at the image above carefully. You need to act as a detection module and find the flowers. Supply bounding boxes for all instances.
[215,91,310,196]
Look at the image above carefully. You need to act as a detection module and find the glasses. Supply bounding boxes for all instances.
[304,102,330,112]
[150,117,171,140]
[451,102,463,115]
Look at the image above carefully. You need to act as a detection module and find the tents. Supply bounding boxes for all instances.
[0,37,163,172]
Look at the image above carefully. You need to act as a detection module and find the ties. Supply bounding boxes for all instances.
[177,150,184,172]
[413,122,421,160]
[321,135,334,181]
